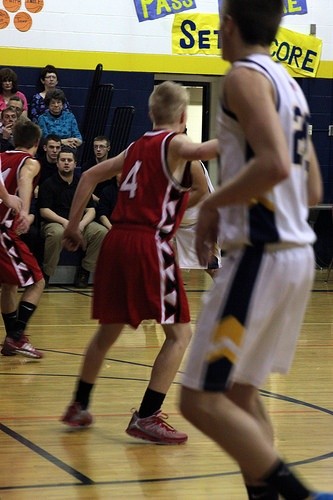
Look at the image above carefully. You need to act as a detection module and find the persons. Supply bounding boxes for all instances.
[0,96,31,153]
[0,121,45,359]
[0,68,27,121]
[61,81,218,445]
[30,65,72,120]
[95,173,123,230]
[38,149,108,289]
[35,89,82,148]
[186,161,223,271]
[34,135,61,199]
[82,136,116,204]
[179,0,333,500]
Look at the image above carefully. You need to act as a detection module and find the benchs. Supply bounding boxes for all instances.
[292,77,333,269]
[0,66,155,283]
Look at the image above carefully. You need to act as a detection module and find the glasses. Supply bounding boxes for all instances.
[92,144,107,149]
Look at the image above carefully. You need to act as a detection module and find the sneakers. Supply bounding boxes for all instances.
[1,333,43,359]
[61,402,93,428]
[125,409,189,444]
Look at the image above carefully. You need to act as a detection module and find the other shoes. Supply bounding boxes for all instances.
[75,268,90,288]
